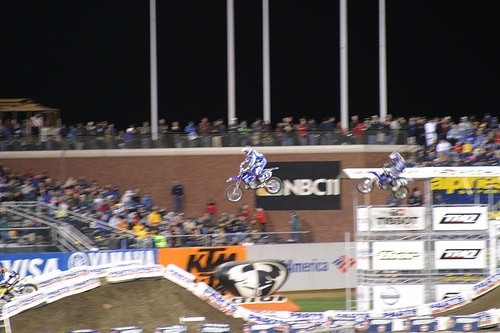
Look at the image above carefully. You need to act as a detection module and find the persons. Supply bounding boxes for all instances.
[240,147,267,183]
[381,151,406,184]
[287,210,301,243]
[4,114,500,167]
[1,167,267,249]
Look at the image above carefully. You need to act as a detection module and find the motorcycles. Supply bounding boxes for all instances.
[224,164,283,203]
[356,164,414,200]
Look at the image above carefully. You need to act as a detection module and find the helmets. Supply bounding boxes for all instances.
[243,146,252,156]
[390,151,400,161]
